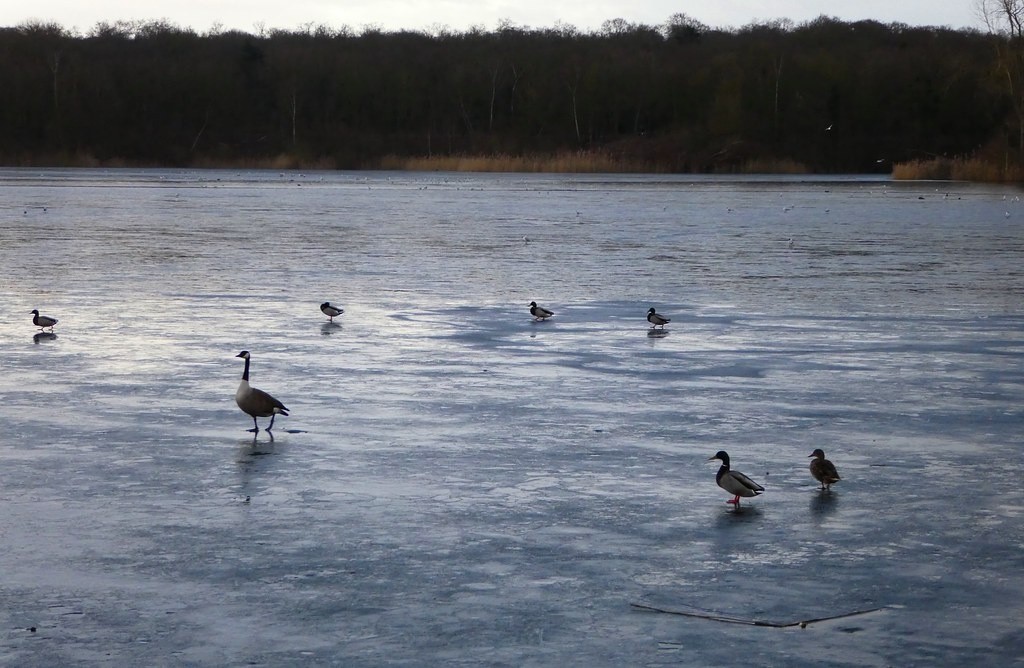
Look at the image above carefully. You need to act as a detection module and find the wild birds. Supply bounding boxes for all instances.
[234,351,289,432]
[29,309,59,331]
[23,172,1023,247]
[807,449,841,490]
[707,451,764,504]
[527,301,555,322]
[319,302,344,321]
[645,307,672,330]
